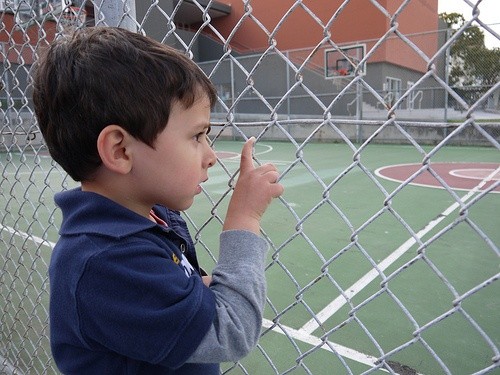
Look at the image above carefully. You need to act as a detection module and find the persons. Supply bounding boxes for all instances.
[33,23,282,375]
